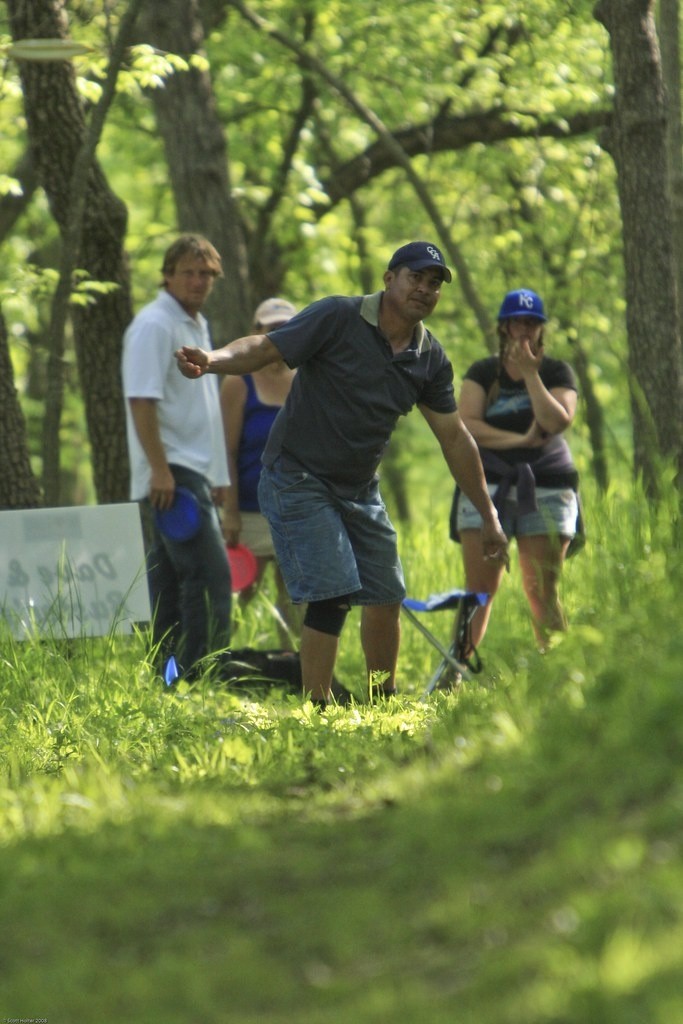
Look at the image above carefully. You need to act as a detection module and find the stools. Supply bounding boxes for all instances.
[402,588,491,697]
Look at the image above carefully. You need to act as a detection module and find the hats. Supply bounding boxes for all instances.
[499,290,547,321]
[254,298,297,325]
[389,242,451,284]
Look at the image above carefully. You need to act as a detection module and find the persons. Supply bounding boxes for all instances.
[118,233,234,689]
[443,287,586,692]
[219,296,308,650]
[172,242,509,718]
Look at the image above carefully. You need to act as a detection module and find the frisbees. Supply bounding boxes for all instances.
[226,543,256,592]
[154,488,203,541]
